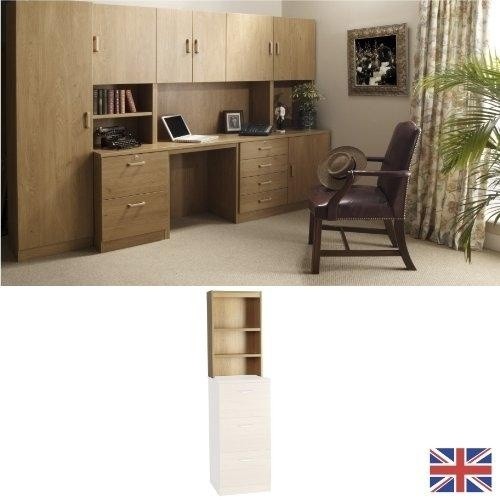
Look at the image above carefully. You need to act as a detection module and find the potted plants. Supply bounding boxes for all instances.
[287,82,326,130]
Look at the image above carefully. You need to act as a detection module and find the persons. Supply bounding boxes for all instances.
[275,103,286,133]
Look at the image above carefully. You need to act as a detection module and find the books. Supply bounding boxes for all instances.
[93,89,137,114]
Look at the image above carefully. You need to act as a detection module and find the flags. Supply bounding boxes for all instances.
[430,448,492,492]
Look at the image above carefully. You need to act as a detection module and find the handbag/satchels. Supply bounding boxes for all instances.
[95,125,125,147]
[101,128,141,149]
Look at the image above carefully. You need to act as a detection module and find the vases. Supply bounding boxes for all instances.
[276,118,287,129]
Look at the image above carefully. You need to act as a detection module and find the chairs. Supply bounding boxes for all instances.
[308,120,422,274]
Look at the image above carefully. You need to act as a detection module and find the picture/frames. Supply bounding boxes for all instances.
[347,23,411,96]
[222,109,244,134]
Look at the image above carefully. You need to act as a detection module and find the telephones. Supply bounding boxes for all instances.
[239,123,273,136]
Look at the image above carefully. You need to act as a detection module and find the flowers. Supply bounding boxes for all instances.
[274,103,289,121]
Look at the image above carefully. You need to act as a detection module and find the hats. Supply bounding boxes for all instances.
[317,145,368,191]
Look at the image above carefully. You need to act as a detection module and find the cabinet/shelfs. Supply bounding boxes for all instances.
[226,12,316,82]
[1,0,94,265]
[157,7,226,84]
[93,147,170,253]
[92,83,157,146]
[206,135,288,225]
[206,290,263,378]
[92,3,156,85]
[209,375,272,496]
[288,130,331,212]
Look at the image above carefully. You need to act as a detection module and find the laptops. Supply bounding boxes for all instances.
[161,114,219,142]
